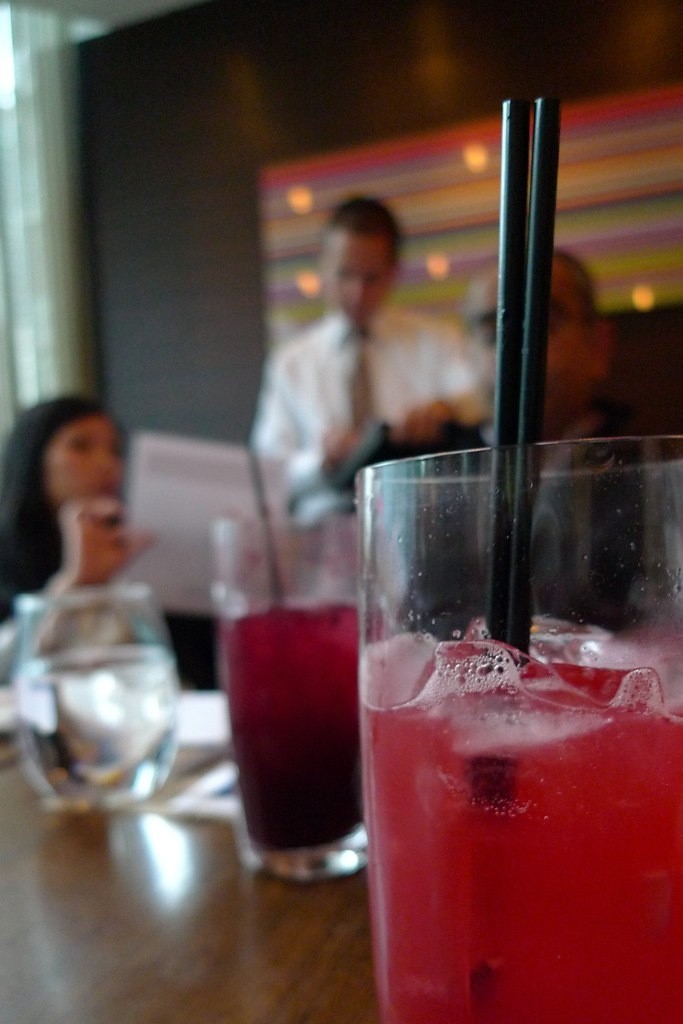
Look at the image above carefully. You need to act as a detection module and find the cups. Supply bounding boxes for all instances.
[353,435,683,1021]
[208,503,368,885]
[6,586,178,813]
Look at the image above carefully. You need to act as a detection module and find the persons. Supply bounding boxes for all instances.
[324,246,649,636]
[244,198,479,507]
[0,392,218,694]
